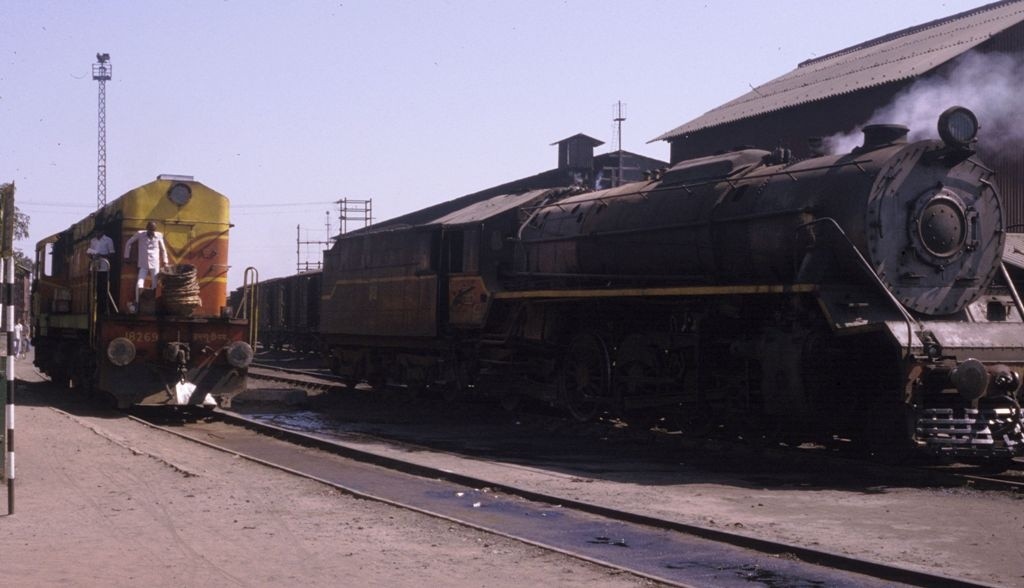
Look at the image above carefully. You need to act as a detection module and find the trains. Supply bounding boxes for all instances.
[230,270,323,354]
[29,174,254,407]
[317,105,1023,461]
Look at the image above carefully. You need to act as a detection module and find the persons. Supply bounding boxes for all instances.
[86,224,115,314]
[21,319,30,352]
[124,221,168,309]
[14,318,23,357]
